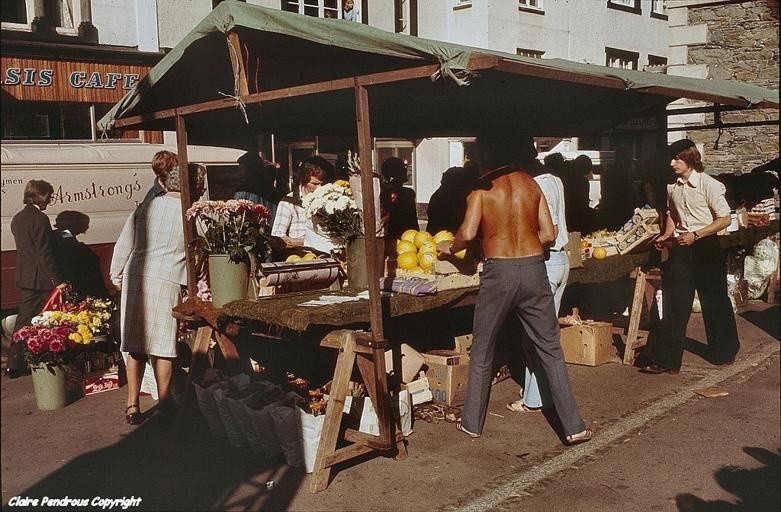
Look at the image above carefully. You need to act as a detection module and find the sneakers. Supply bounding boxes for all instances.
[124,404,142,426]
[505,395,553,414]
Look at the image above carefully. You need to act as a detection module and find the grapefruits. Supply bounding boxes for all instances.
[386,229,465,275]
[593,247,607,260]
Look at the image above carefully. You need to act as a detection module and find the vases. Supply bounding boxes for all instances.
[29,361,70,412]
[345,238,386,293]
[207,256,251,310]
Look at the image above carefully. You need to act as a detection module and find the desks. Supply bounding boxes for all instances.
[171,249,663,493]
[716,215,781,307]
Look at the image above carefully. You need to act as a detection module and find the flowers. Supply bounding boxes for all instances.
[298,147,395,243]
[191,361,329,475]
[12,293,122,369]
[186,199,271,262]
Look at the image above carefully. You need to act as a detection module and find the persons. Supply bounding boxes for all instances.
[638,139,739,374]
[142,152,178,201]
[425,128,666,237]
[231,153,420,267]
[437,138,592,444]
[6,180,75,378]
[342,0,357,23]
[506,141,570,412]
[110,164,207,424]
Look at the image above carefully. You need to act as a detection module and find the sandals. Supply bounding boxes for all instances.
[565,427,594,445]
[454,419,482,440]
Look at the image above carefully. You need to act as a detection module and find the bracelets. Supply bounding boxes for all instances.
[450,244,453,253]
[693,231,699,241]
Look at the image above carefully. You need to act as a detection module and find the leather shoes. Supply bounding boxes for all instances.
[4,365,31,380]
[640,359,682,376]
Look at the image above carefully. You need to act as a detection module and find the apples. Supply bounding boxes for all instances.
[581,241,588,249]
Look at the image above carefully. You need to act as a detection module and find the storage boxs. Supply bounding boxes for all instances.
[564,231,583,269]
[321,379,413,438]
[67,369,120,398]
[413,332,474,408]
[451,330,512,385]
[555,320,614,367]
